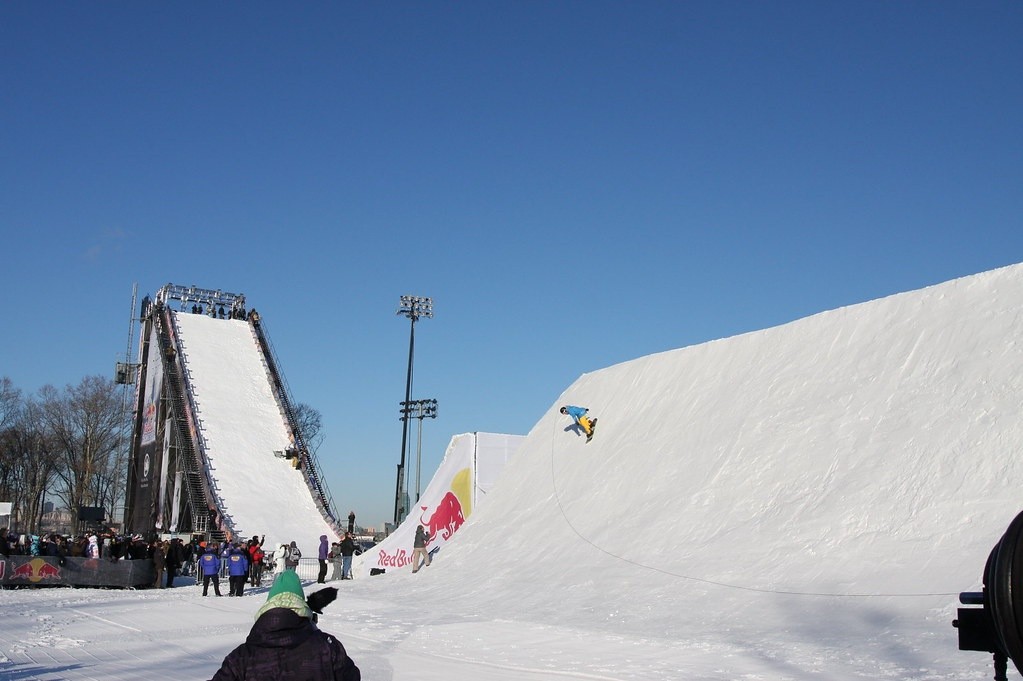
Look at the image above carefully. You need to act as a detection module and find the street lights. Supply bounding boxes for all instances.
[398,397,438,504]
[393,293,435,530]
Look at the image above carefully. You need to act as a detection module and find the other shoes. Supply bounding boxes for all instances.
[342,577,350,580]
[412,570,416,573]
[426,565,429,566]
[587,431,593,438]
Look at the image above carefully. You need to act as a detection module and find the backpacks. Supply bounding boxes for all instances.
[288,548,300,562]
[328,551,339,563]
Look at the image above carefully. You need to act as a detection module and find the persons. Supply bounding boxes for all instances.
[157,294,164,316]
[348,512,355,533]
[208,506,217,529]
[331,532,356,579]
[192,304,216,318]
[219,306,225,319]
[560,405,593,437]
[249,308,258,329]
[228,309,232,319]
[291,448,303,469]
[274,541,301,581]
[163,345,178,364]
[0,527,265,597]
[234,309,246,320]
[412,525,430,573]
[207,569,361,681]
[318,535,328,583]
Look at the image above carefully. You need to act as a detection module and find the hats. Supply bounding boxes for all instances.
[267,570,305,601]
[560,407,567,414]
[233,543,241,549]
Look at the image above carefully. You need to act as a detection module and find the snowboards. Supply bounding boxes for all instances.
[586,418,597,443]
